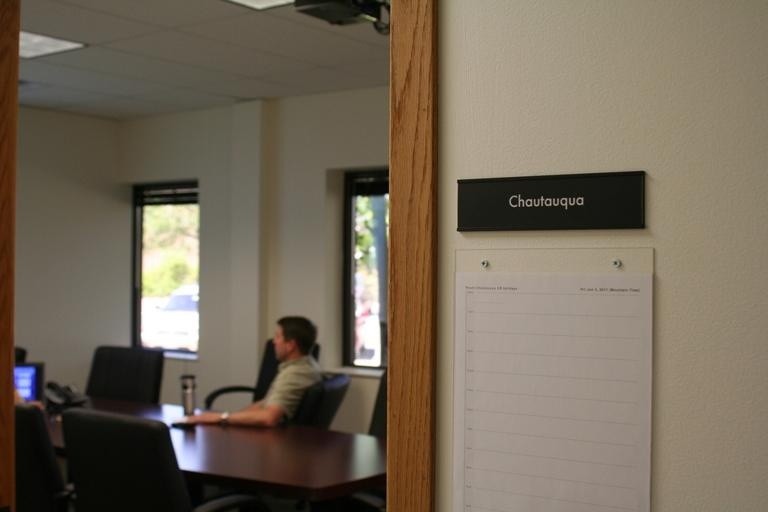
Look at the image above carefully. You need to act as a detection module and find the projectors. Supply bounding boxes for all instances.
[292,0,381,27]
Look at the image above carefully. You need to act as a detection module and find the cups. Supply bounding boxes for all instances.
[180,374,196,414]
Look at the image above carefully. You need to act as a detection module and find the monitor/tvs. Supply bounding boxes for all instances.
[13,363,45,402]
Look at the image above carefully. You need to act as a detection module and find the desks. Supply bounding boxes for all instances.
[44,395,386,512]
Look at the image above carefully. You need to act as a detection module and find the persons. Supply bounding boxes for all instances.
[170,317,325,428]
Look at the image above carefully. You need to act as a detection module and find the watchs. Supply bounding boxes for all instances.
[220,409,231,426]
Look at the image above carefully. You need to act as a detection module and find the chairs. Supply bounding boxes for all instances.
[204,335,353,432]
[84,345,167,404]
[12,399,258,512]
